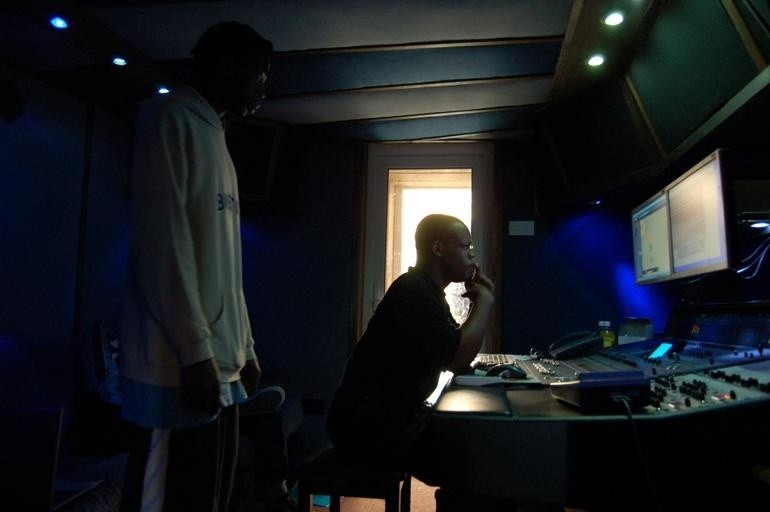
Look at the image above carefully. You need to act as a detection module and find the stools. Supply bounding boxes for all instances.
[298,446,413,512]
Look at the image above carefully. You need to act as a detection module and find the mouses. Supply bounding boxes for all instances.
[486,364,528,379]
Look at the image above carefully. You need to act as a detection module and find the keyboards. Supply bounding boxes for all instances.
[478,353,518,370]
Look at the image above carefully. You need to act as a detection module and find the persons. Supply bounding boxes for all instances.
[113,21,274,510]
[325,214,496,511]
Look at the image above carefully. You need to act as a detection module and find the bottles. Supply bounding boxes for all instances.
[596,317,616,349]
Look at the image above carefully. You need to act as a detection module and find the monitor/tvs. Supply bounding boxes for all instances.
[665,148,740,281]
[630,189,673,285]
